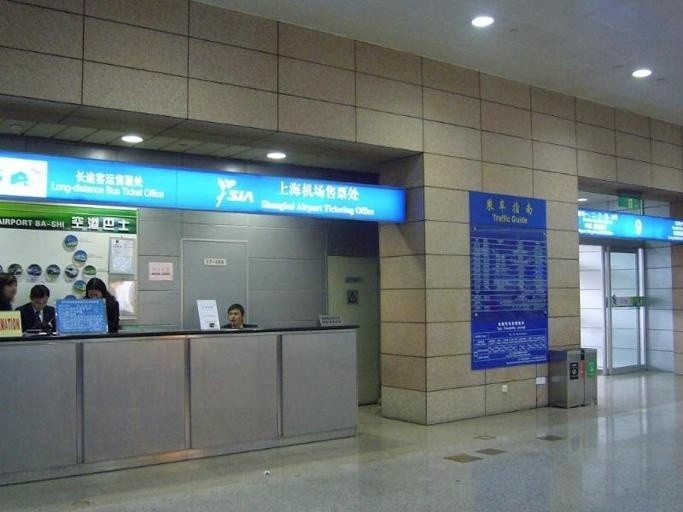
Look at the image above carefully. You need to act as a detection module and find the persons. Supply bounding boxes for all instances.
[0,274,17,312]
[220,303,250,329]
[83,277,120,332]
[14,284,55,333]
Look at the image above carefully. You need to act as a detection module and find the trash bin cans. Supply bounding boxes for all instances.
[548,348,597,408]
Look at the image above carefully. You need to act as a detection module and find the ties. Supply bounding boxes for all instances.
[35,311,41,326]
